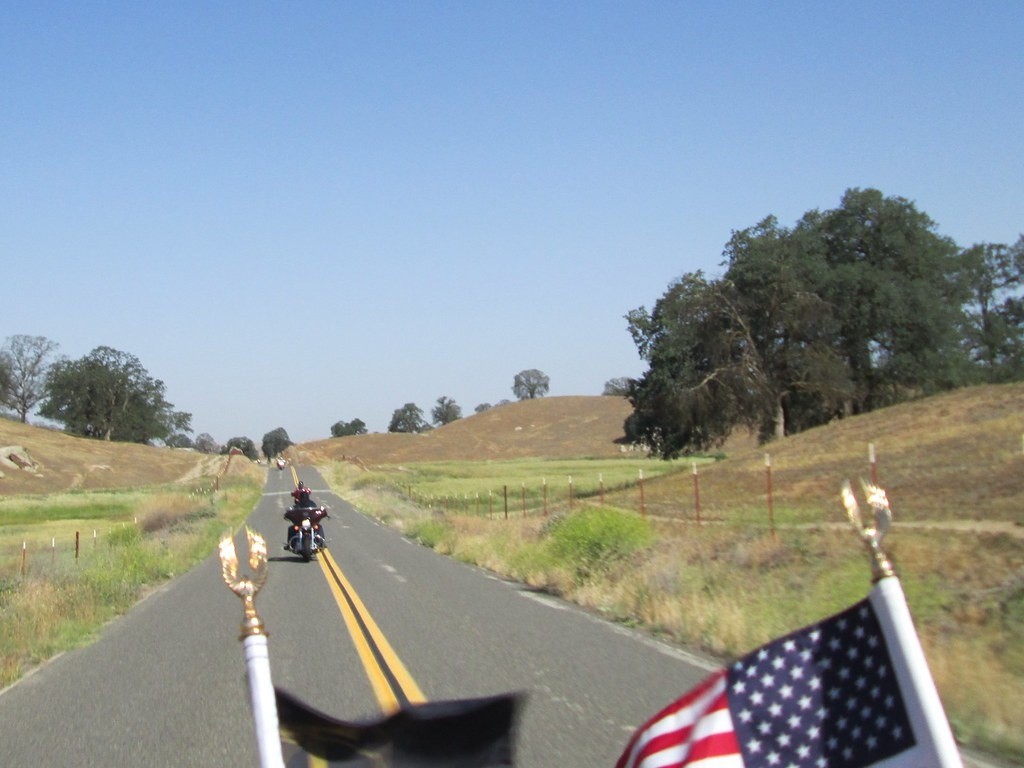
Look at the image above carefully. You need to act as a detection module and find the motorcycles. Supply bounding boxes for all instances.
[283,506,329,562]
[277,459,285,470]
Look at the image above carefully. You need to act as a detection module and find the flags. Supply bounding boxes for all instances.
[243,668,531,768]
[614,589,927,768]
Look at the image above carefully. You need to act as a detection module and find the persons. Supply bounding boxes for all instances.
[291,481,312,504]
[283,492,327,551]
[254,455,290,470]
